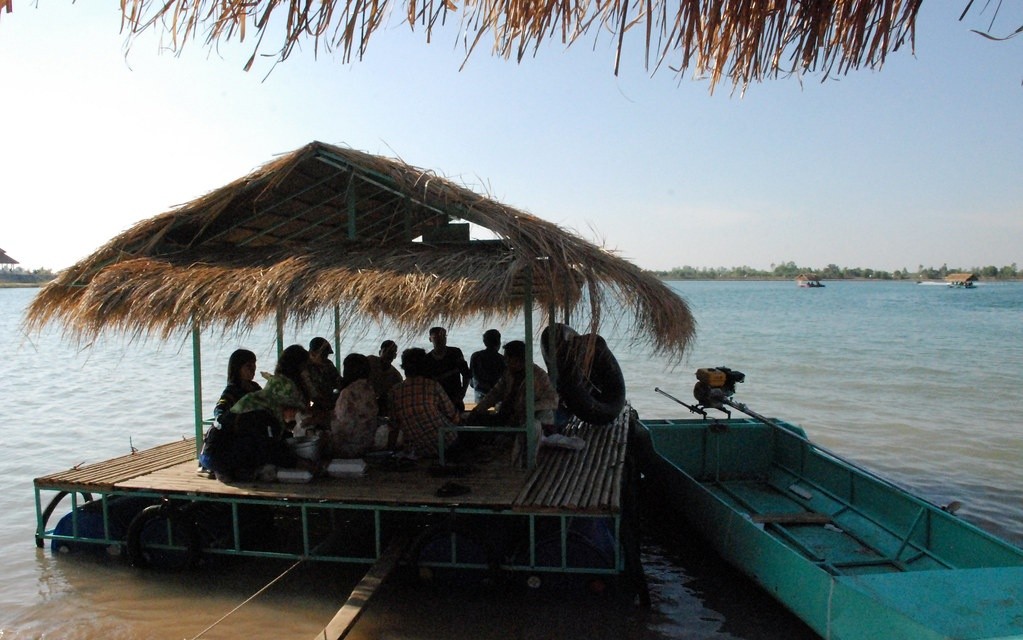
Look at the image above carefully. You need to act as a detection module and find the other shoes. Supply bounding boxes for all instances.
[436,483,469,497]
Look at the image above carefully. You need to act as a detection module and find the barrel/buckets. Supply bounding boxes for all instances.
[291,428,321,463]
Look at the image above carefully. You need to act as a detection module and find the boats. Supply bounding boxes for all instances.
[945,271,980,289]
[797,273,828,289]
[633,367,1023,640]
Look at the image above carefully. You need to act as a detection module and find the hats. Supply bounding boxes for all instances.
[310,337,333,354]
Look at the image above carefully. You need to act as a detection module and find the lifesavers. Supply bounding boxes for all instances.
[552,334,627,428]
[127,503,206,578]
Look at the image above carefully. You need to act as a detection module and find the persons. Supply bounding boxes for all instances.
[264,343,316,421]
[469,341,560,443]
[470,330,507,399]
[199,375,328,484]
[324,353,378,453]
[303,338,340,400]
[364,355,402,394]
[379,341,396,365]
[214,349,270,418]
[384,349,459,461]
[425,327,470,412]
